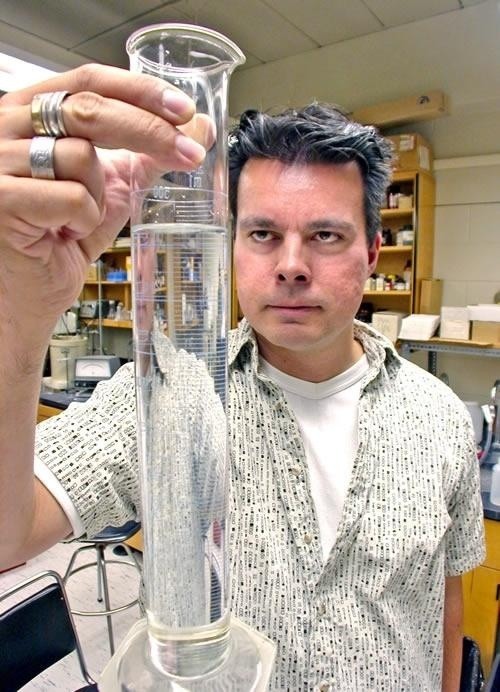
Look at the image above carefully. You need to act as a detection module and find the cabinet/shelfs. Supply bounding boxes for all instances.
[355,173,434,330]
[399,341,500,520]
[82,187,239,328]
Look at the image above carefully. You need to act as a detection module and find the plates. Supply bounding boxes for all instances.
[42,375,72,391]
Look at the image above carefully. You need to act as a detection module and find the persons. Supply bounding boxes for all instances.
[0,62,487,692]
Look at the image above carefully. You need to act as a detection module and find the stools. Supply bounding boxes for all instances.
[60,521,142,656]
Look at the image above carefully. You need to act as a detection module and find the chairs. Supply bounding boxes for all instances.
[1,569,99,692]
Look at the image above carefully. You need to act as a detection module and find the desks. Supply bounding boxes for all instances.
[40,390,500,687]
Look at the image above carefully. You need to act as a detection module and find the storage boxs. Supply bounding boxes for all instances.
[385,133,432,174]
[439,306,470,341]
[371,310,409,345]
[398,313,440,341]
[466,304,500,321]
[470,320,500,348]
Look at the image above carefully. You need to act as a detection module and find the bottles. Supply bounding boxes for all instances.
[100,23,277,691]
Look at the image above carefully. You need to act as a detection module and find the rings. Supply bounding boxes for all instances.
[29,135,55,180]
[31,90,69,138]
